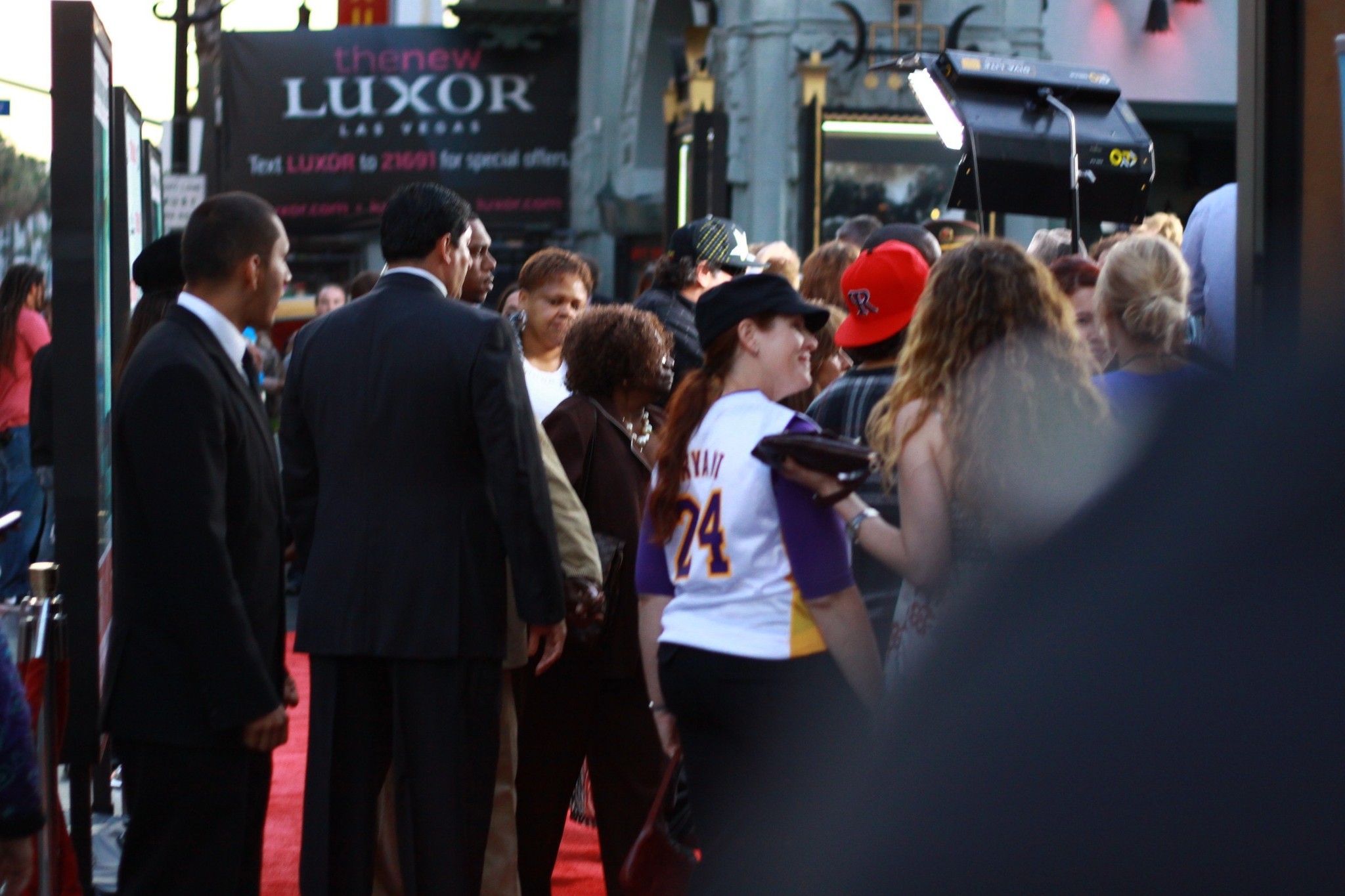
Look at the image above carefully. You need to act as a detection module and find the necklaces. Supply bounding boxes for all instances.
[618,407,654,453]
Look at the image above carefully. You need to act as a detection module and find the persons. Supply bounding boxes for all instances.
[450,184,1345,895]
[0,625,49,896]
[103,191,380,896]
[0,263,57,601]
[280,181,563,895]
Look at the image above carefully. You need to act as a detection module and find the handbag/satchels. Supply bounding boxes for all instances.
[618,748,699,896]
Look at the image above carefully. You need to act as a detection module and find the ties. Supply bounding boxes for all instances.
[243,352,262,406]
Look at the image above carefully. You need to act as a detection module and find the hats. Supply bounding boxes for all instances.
[670,217,771,268]
[693,274,831,343]
[921,220,980,251]
[833,240,931,348]
[861,223,943,266]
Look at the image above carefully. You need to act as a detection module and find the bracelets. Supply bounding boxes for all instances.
[844,506,879,540]
[649,703,668,712]
[819,488,854,506]
